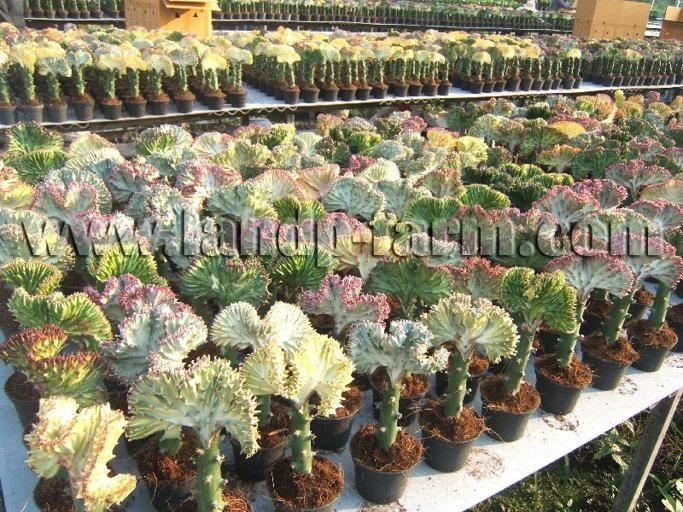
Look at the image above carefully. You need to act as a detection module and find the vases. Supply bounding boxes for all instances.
[309,386,367,445]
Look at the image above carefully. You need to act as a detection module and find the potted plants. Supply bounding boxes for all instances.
[1,89,682,404]
[392,22,584,95]
[243,335,357,510]
[567,33,683,87]
[351,319,449,503]
[129,355,260,509]
[533,252,630,415]
[421,293,516,471]
[220,26,450,107]
[0,27,250,128]
[26,395,138,510]
[483,268,579,442]
